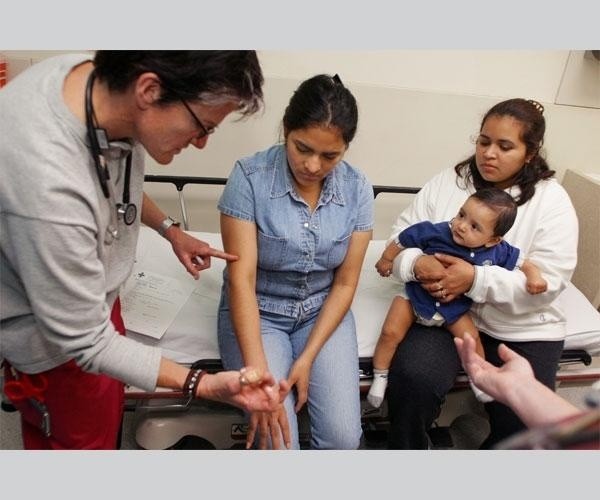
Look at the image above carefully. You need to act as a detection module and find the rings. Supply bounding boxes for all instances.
[239,376,249,387]
[439,291,447,300]
[435,282,443,291]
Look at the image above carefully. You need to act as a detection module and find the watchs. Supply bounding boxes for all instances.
[158,216,182,240]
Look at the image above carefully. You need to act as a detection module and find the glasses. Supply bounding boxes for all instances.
[179,96,216,142]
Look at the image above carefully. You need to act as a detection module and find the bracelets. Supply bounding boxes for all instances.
[183,368,208,401]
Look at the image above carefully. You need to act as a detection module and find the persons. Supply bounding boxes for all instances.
[366,185,548,409]
[452,329,600,449]
[387,97,579,449]
[217,73,375,449]
[0,50,293,449]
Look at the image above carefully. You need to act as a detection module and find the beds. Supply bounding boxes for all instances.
[123,170,599,450]
